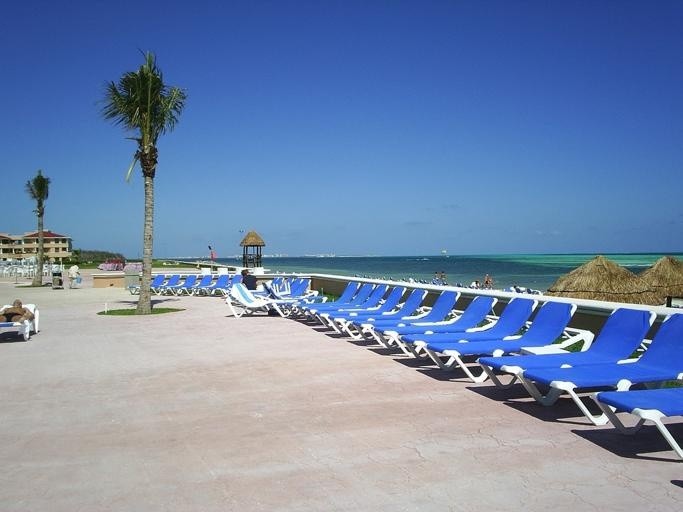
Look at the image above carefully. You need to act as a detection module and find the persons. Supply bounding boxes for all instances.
[1,299,34,324]
[241,269,256,290]
[486,278,492,289]
[469,280,480,288]
[68,263,80,287]
[483,272,489,283]
[513,285,521,293]
[441,271,446,284]
[434,272,438,280]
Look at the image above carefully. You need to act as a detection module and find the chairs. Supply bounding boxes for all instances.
[220,276,328,320]
[0,258,81,289]
[127,275,242,298]
[0,304,39,340]
